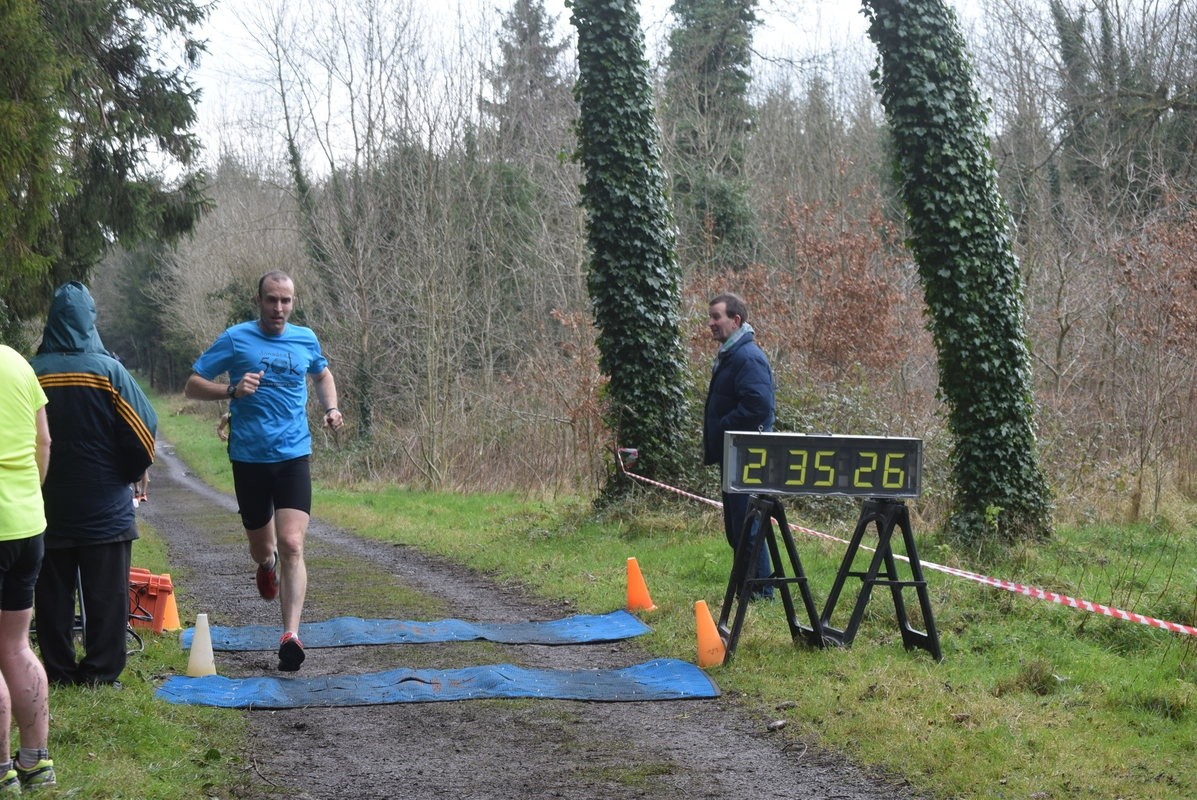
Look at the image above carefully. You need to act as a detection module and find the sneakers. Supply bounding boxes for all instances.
[0,769,21,796]
[12,751,56,788]
[141,494,147,501]
[135,491,141,503]
[256,552,280,602]
[278,632,306,672]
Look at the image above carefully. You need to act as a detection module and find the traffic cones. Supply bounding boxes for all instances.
[695,599,726,669]
[160,573,183,630]
[627,557,658,613]
[184,614,217,677]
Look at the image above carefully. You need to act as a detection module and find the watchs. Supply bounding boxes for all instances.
[227,384,238,400]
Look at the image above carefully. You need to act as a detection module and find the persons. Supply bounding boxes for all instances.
[185,271,344,672]
[703,295,775,601]
[217,411,240,514]
[134,468,148,501]
[30,280,157,691]
[0,343,56,796]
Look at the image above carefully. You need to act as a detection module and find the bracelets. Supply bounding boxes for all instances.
[325,407,337,414]
